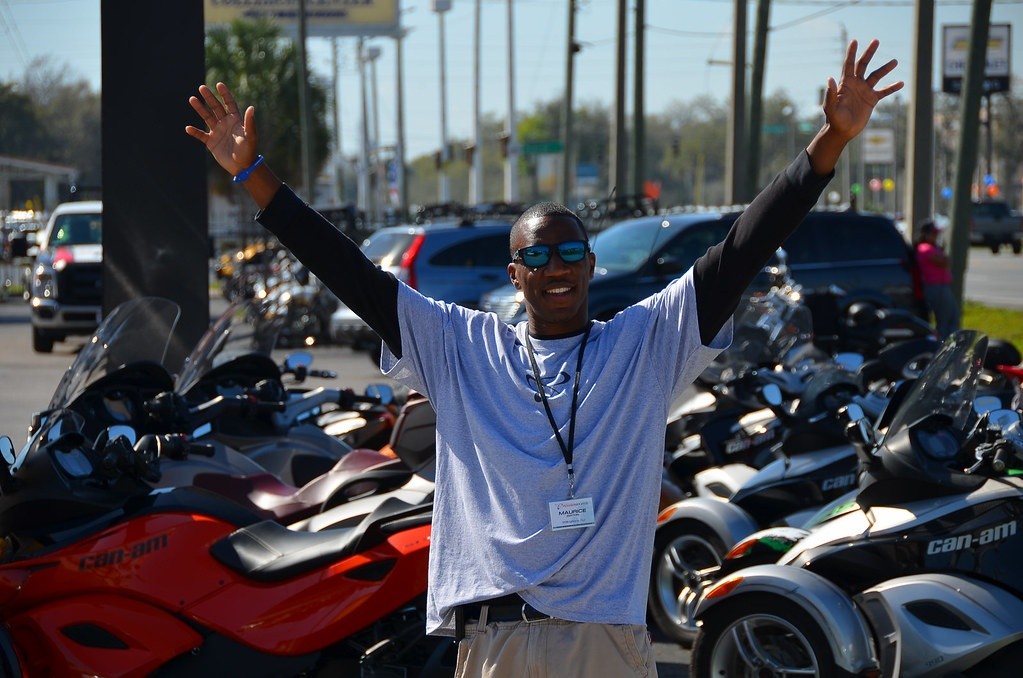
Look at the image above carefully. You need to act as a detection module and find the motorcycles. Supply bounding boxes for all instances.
[0,244,1021,678]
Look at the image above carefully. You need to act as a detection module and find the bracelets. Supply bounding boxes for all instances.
[232,154,265,185]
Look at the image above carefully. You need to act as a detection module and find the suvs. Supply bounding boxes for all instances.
[330,218,516,355]
[26,199,105,355]
[585,207,932,360]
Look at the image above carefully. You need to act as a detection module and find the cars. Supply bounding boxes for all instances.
[317,191,662,236]
[959,196,1023,254]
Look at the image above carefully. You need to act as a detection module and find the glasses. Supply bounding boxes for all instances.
[511,239,591,270]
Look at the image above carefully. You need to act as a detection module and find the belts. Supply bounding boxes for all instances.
[453,600,554,623]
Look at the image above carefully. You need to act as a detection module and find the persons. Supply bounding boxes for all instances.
[916,223,960,342]
[185,40,905,678]
